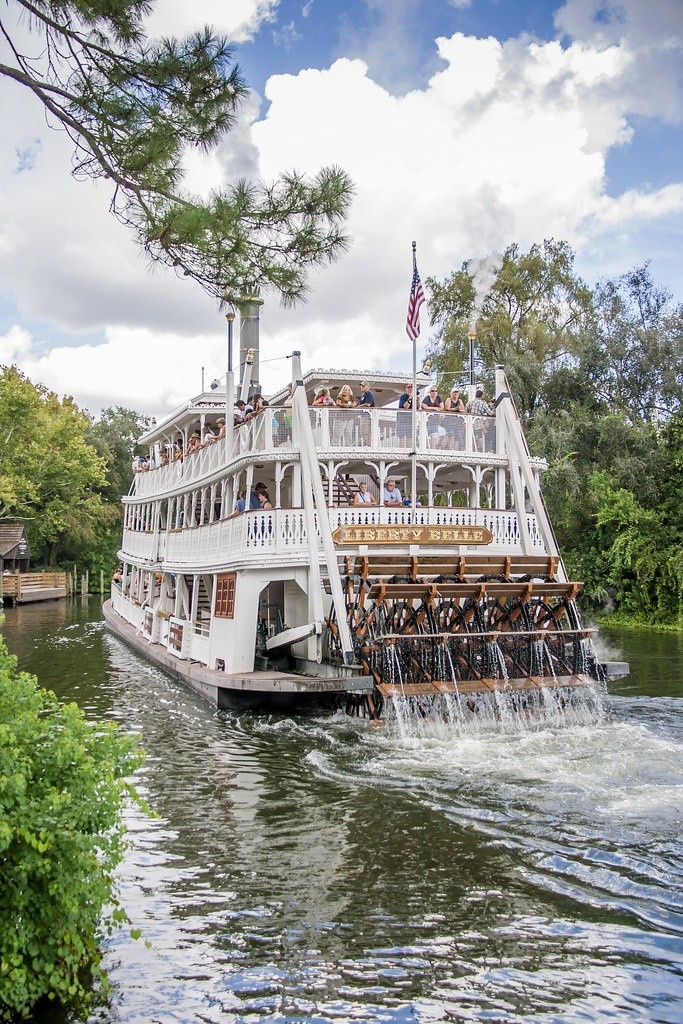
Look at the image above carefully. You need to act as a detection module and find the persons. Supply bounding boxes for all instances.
[355,381,375,446]
[421,386,444,450]
[383,480,402,507]
[353,482,376,507]
[466,391,496,454]
[132,394,278,477]
[444,388,464,451]
[283,383,292,443]
[312,388,335,426]
[396,384,420,449]
[331,385,356,447]
[219,481,273,545]
[403,494,421,507]
[112,511,185,590]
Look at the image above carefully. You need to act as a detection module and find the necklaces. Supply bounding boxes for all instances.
[260,499,267,507]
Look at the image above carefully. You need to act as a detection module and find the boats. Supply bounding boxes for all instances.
[103,242,631,722]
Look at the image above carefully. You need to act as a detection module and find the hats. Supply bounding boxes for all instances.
[191,434,200,438]
[451,388,459,393]
[476,390,483,397]
[256,482,267,489]
[235,400,244,406]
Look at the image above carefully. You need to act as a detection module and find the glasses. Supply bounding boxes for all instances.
[387,484,395,487]
[432,390,438,392]
[453,391,458,393]
[359,383,367,387]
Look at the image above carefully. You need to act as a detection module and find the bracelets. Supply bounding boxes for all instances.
[407,400,411,404]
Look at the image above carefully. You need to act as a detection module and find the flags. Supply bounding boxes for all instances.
[406,259,424,341]
